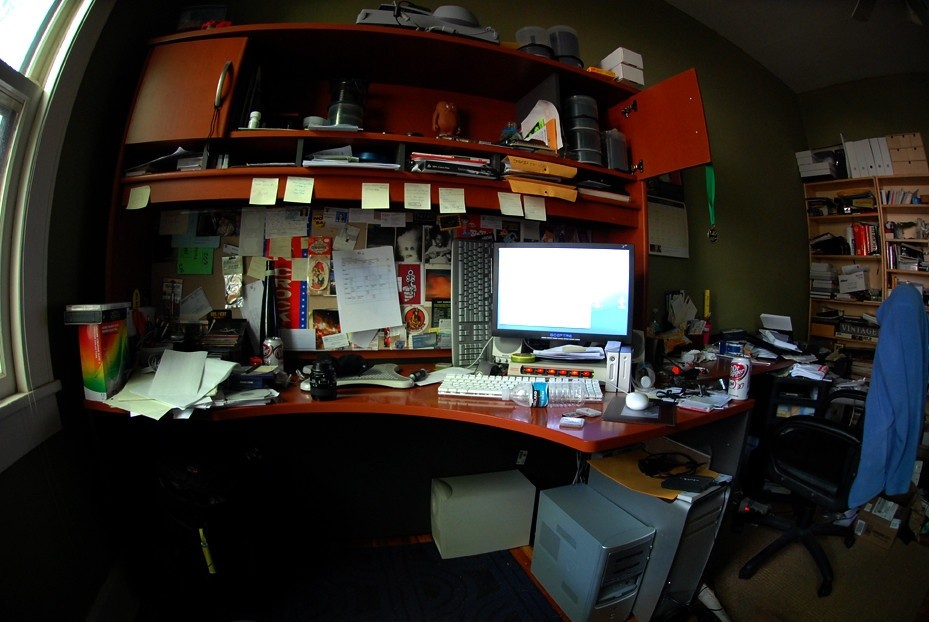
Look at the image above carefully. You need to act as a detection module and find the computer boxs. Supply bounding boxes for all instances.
[586,464,731,622]
[528,481,656,622]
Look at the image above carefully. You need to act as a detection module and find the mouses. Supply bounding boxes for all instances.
[626,392,649,410]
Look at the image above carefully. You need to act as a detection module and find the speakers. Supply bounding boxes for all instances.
[491,334,523,363]
[430,469,536,559]
[630,330,644,364]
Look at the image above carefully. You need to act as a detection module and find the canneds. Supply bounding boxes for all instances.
[263,337,284,371]
[727,356,751,401]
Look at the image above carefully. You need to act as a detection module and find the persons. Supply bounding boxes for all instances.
[338,213,344,223]
[425,232,451,264]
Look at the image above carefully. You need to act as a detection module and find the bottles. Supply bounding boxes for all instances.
[502,382,585,407]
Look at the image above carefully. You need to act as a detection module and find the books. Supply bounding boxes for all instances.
[579,172,631,203]
[201,319,247,347]
[810,217,882,300]
[884,242,899,272]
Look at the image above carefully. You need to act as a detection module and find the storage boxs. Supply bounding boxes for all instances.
[856,496,907,550]
[838,271,869,293]
[599,46,644,89]
[794,133,929,178]
[810,323,836,337]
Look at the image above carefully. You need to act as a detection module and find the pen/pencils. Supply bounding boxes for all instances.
[244,365,260,375]
[144,314,171,337]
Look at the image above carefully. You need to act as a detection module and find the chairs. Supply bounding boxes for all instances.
[732,283,929,597]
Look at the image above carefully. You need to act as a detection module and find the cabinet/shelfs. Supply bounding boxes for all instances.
[78,20,753,622]
[793,175,929,380]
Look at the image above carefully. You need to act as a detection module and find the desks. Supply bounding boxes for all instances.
[674,339,828,380]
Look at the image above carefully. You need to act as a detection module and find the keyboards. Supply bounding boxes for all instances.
[438,373,604,403]
[451,238,496,366]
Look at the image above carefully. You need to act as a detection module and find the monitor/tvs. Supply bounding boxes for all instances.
[492,243,635,360]
